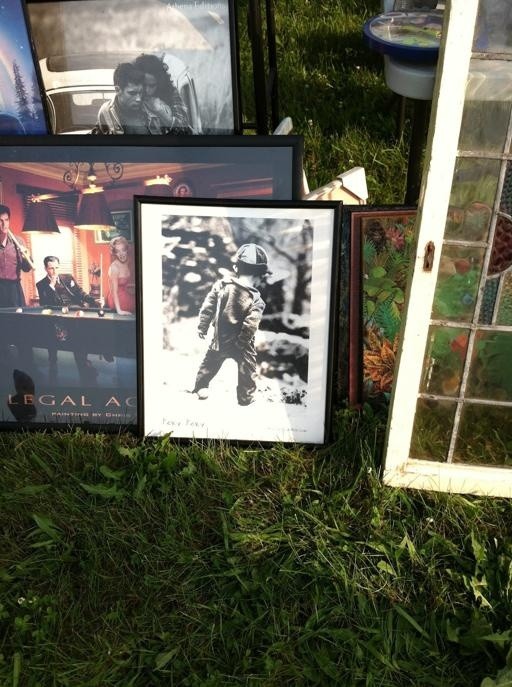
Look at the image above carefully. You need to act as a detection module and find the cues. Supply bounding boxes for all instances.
[8,229,35,271]
[100,253,103,307]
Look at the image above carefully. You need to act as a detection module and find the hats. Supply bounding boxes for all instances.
[235,243,268,266]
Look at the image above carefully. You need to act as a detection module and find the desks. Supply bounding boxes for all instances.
[0,305,136,379]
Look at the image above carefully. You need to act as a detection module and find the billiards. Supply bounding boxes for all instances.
[42,309,51,314]
[76,310,83,316]
[17,309,22,313]
[62,307,68,313]
[99,310,104,317]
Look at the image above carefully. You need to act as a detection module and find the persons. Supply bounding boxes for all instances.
[171,181,193,197]
[105,235,136,316]
[132,55,197,134]
[35,256,105,311]
[0,204,34,309]
[184,244,273,406]
[91,62,171,135]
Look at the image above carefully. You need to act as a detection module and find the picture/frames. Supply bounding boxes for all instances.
[132,194,343,445]
[0,134,300,434]
[21,0,243,135]
[340,205,492,419]
[94,210,132,243]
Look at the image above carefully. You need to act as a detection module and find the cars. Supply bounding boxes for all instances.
[39,50,202,135]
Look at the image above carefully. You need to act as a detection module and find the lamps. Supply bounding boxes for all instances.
[63,162,123,230]
[22,201,61,235]
